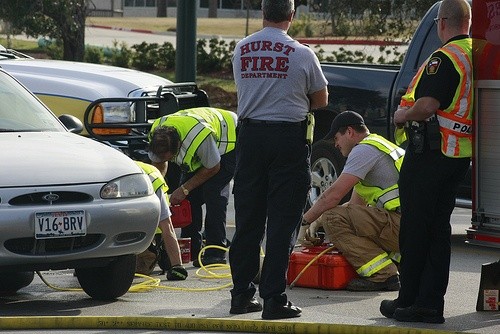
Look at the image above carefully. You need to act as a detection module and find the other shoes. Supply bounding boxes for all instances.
[229,296,262,314]
[261,301,302,319]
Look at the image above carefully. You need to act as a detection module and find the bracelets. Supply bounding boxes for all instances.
[302,218,310,227]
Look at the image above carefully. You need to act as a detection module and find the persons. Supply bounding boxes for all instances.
[379,0,475,322]
[151,108,238,268]
[298,111,409,291]
[137,157,189,281]
[229,0,333,317]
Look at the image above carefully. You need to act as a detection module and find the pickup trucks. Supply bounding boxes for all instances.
[301,0,473,223]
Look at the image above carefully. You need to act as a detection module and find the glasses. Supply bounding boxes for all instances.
[434,16,448,24]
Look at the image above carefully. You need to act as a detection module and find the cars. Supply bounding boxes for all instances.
[0,65,161,300]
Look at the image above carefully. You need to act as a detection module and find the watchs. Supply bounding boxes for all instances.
[180,184,189,195]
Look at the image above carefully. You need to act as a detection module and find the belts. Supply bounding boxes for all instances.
[238,119,295,127]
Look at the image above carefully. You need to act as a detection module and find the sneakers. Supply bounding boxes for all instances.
[346,274,402,291]
[380,296,444,323]
[193,255,226,267]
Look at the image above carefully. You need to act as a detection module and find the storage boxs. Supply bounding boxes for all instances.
[287,252,357,291]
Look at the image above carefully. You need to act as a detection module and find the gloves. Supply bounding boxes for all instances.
[166,264,188,281]
[298,219,321,247]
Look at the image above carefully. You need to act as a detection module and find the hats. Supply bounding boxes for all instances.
[324,111,364,140]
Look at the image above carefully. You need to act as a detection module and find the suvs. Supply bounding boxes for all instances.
[0,44,211,165]
